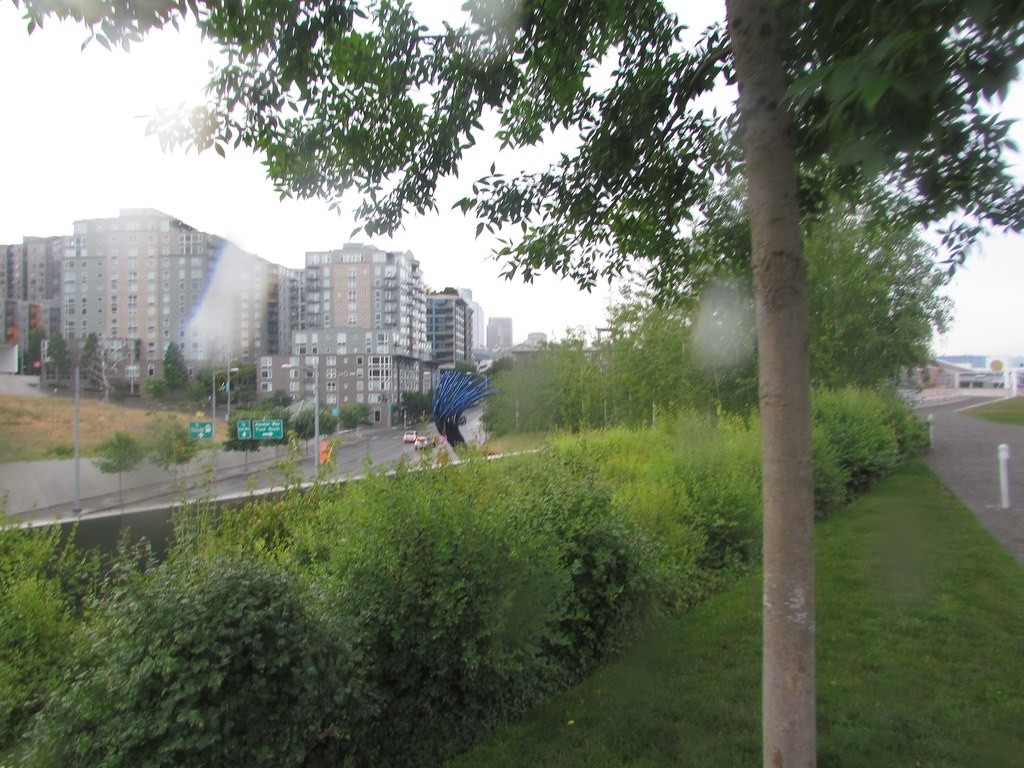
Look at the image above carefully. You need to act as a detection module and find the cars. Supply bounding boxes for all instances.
[403,430,417,444]
[413,436,428,450]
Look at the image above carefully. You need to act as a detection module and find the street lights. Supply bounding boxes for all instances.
[281,362,319,480]
[211,368,240,455]
